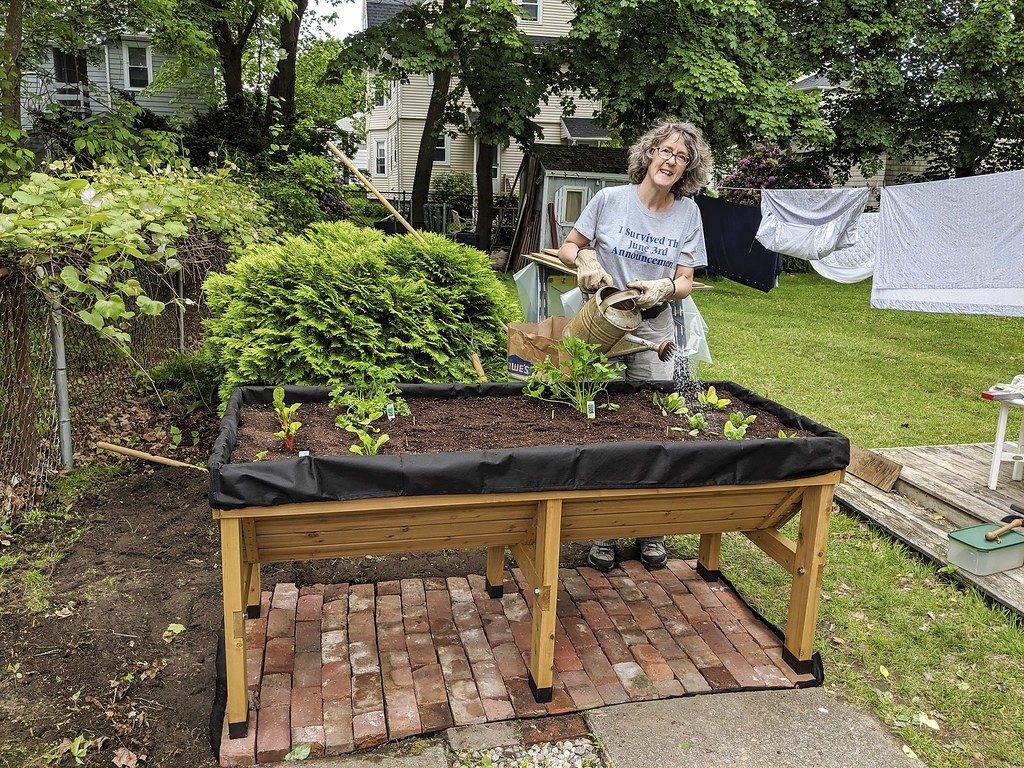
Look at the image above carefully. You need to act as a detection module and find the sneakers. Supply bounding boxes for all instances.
[588,544,616,571]
[638,539,667,567]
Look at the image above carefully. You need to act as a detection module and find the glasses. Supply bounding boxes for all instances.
[653,146,690,166]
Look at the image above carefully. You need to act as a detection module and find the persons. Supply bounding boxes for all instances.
[558,123,713,570]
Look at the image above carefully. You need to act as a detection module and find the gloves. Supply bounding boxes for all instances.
[626,277,675,309]
[574,247,614,290]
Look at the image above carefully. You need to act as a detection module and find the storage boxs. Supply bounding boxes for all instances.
[947,523,1024,576]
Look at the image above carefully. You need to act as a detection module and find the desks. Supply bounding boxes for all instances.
[519,251,714,384]
